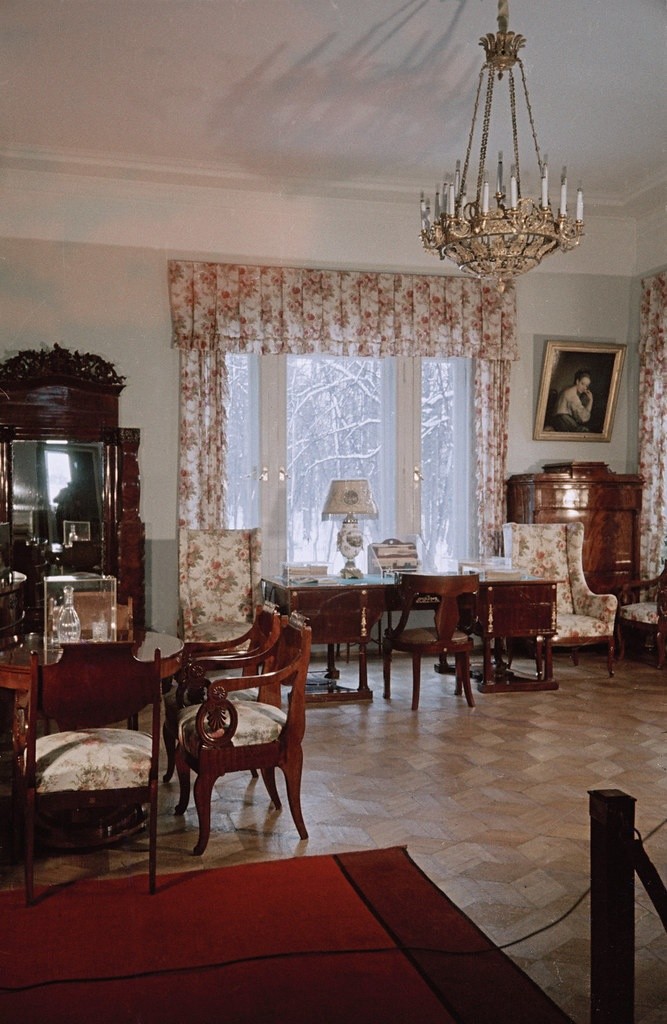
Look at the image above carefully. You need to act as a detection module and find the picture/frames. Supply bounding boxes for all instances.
[533,341,628,443]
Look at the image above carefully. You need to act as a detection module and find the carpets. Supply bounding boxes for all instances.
[0,847,576,1024]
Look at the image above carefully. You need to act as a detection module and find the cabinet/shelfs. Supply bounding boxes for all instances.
[505,462,645,659]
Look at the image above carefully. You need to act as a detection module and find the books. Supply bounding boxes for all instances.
[458,562,521,581]
[283,565,337,584]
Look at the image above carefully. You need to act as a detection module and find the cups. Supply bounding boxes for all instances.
[92,621,108,643]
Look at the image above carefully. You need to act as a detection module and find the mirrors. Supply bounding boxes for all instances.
[12,440,107,572]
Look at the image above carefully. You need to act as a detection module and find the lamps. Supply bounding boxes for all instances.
[322,479,377,579]
[417,1,583,295]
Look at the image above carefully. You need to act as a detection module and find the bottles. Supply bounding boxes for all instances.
[57,587,80,645]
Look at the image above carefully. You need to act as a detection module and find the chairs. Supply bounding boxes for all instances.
[616,563,666,668]
[10,640,163,905]
[178,526,265,652]
[173,614,312,854]
[162,606,280,799]
[502,521,619,675]
[383,569,481,711]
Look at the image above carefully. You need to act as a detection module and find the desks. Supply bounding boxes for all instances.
[263,575,564,702]
[0,633,186,848]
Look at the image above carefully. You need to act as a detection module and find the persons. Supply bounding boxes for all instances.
[54,482,82,541]
